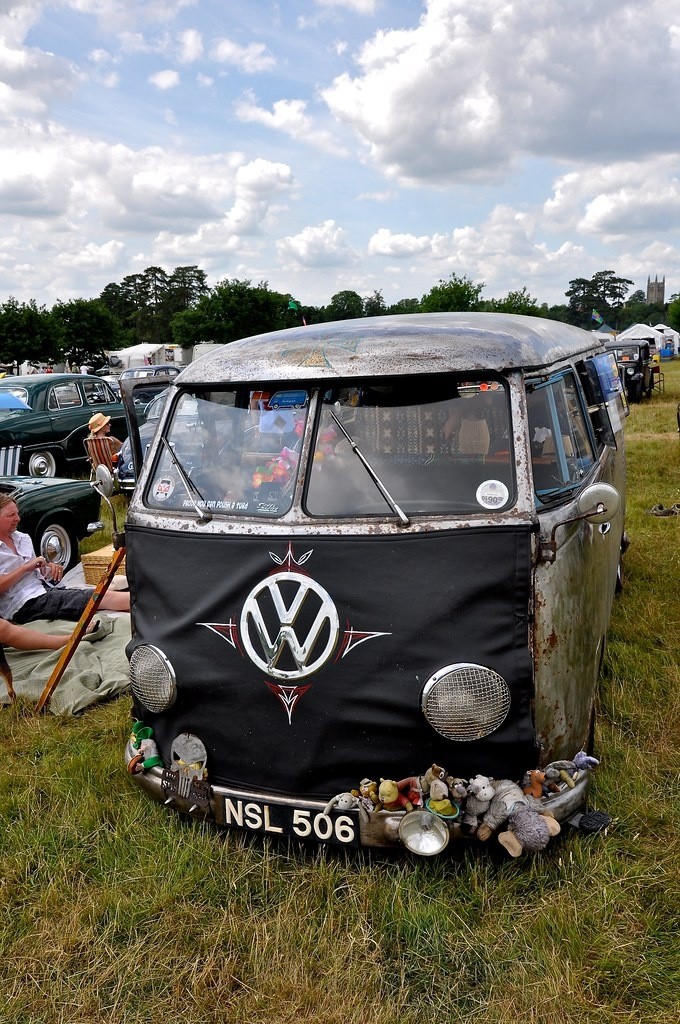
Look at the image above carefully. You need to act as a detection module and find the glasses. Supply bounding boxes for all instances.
[105,422,109,426]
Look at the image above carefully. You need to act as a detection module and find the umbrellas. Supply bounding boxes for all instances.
[0,392,33,411]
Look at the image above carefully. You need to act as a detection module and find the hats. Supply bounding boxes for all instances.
[88,413,111,434]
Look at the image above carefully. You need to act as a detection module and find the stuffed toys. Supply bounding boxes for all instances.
[127,720,163,771]
[323,751,599,857]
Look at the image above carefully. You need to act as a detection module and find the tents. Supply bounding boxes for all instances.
[108,344,166,371]
[595,322,680,362]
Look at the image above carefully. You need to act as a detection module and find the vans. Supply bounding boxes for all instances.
[84,309,631,858]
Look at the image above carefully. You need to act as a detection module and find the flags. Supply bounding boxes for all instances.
[592,309,603,324]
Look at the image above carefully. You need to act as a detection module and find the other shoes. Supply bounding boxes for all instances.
[649,503,670,515]
[84,619,100,635]
[668,503,680,514]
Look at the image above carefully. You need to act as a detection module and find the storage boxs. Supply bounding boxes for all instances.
[82,543,127,586]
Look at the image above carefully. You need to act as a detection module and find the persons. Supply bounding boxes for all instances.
[250,391,297,453]
[89,413,123,469]
[443,390,568,458]
[0,618,100,650]
[0,493,130,625]
[31,361,94,374]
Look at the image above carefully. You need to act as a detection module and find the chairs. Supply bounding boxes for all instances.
[84,438,120,478]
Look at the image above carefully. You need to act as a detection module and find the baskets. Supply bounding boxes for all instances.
[81,542,126,586]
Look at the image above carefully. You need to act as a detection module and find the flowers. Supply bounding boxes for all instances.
[244,418,333,496]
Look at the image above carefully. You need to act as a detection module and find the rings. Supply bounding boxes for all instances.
[59,571,61,572]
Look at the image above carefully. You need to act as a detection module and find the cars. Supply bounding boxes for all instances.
[60,363,257,498]
[0,474,106,585]
[0,372,154,478]
[592,332,655,404]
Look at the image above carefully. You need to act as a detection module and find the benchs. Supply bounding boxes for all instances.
[353,398,570,497]
